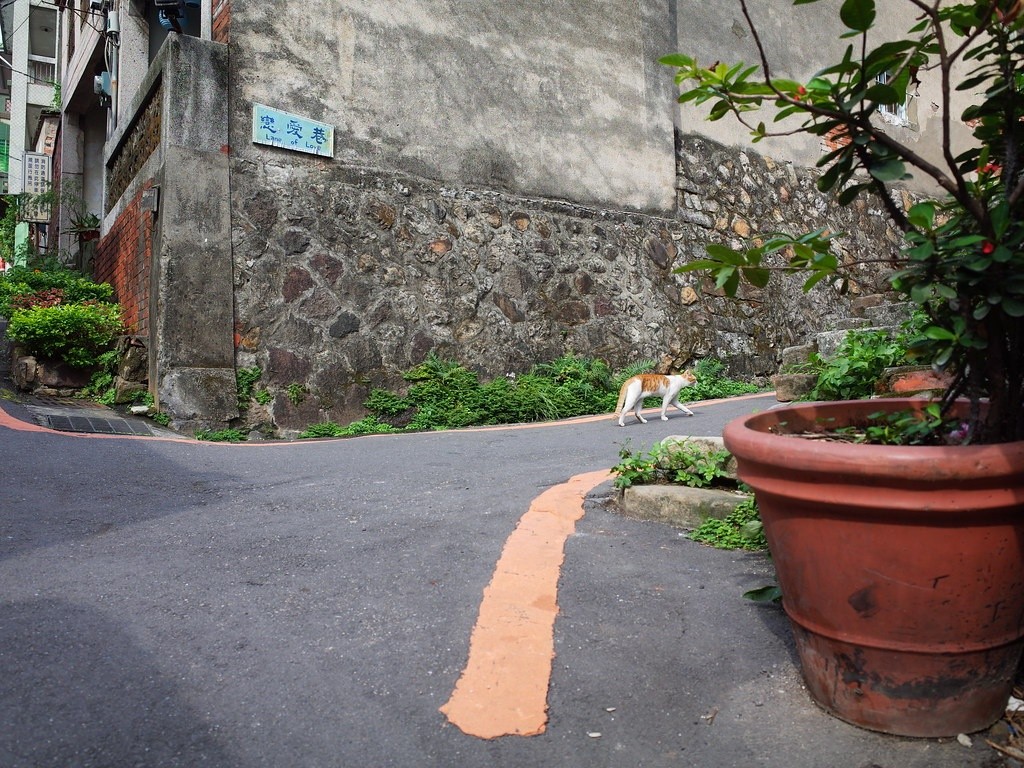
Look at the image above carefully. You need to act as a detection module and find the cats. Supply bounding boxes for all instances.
[612,369,698,427]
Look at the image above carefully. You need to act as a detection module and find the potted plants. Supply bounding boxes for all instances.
[657,1,1024,745]
[65,212,101,248]
